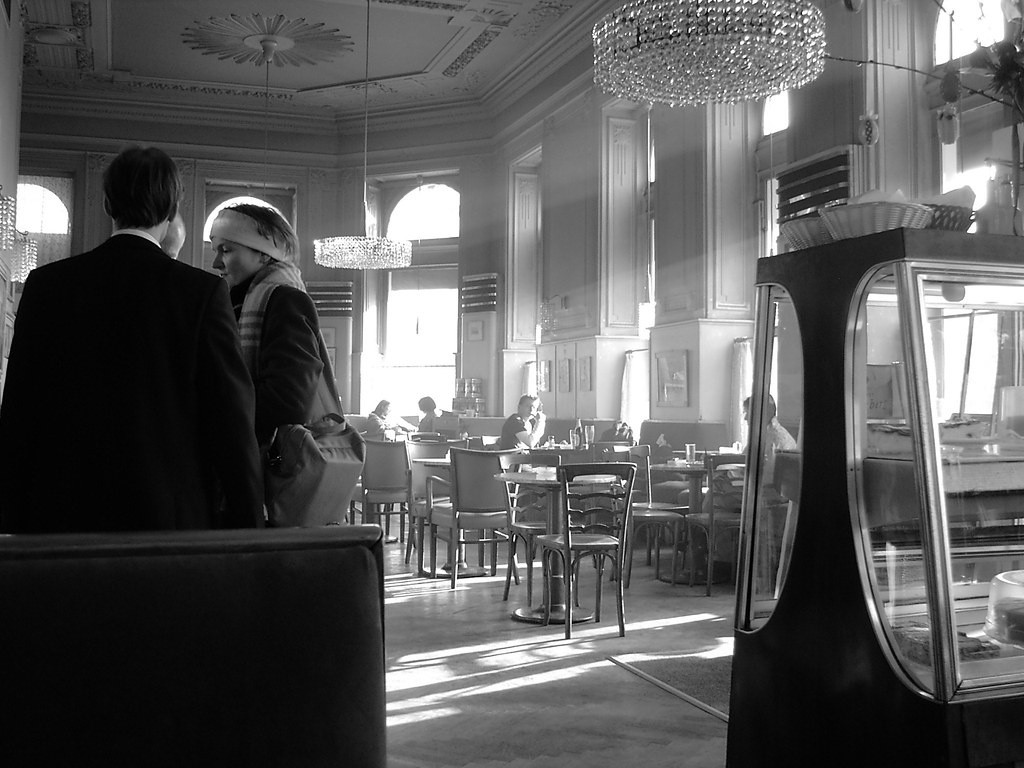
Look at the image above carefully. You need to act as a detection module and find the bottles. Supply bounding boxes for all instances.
[977,176,1021,236]
[575,417,583,447]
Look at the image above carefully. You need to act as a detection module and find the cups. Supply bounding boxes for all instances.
[569,430,577,448]
[585,425,595,444]
[548,435,555,449]
[684,443,696,465]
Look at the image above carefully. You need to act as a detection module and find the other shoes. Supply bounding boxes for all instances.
[657,569,705,586]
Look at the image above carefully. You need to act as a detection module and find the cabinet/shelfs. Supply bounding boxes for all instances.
[726,226,1024,768]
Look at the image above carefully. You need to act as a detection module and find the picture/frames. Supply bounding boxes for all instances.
[467,320,483,340]
[327,347,336,374]
[558,359,570,393]
[655,348,689,407]
[320,326,337,347]
[578,356,591,392]
[540,360,551,393]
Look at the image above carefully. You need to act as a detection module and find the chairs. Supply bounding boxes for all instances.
[349,417,799,639]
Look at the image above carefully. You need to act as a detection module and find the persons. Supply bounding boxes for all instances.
[415,396,438,432]
[367,399,403,438]
[160,211,186,260]
[1,143,268,534]
[500,393,546,452]
[673,391,797,583]
[210,203,324,491]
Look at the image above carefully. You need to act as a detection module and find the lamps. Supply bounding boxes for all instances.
[1,184,16,250]
[12,231,38,283]
[314,0,412,270]
[591,0,829,107]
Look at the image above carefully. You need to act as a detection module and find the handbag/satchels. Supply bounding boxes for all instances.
[263,329,367,529]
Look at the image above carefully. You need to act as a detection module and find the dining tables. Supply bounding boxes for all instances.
[414,458,489,577]
[530,440,589,468]
[495,470,617,622]
[650,461,745,585]
